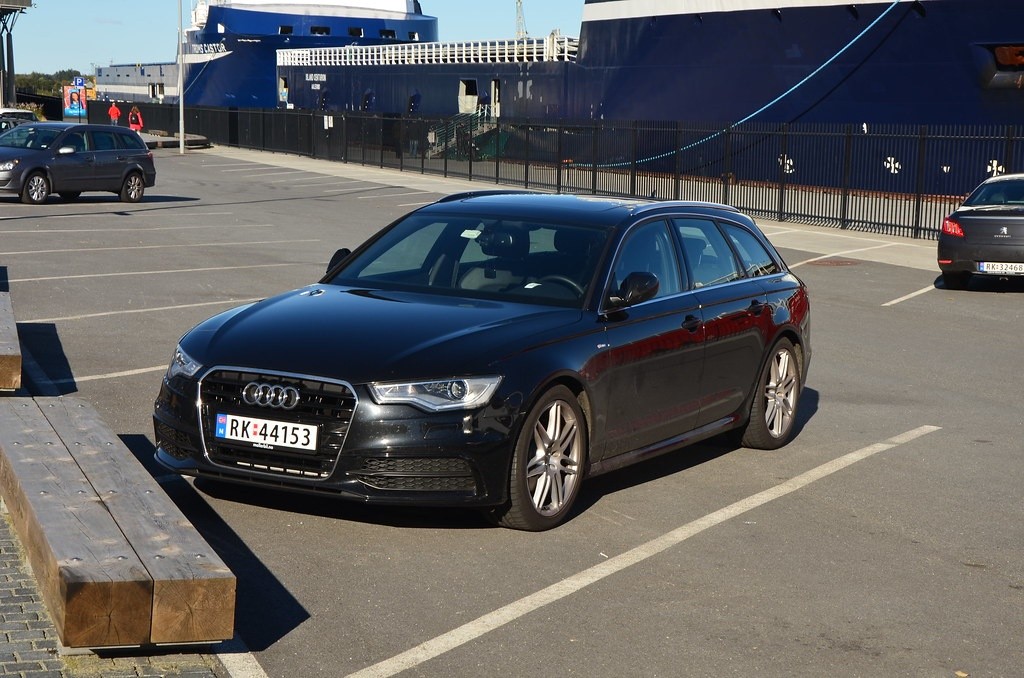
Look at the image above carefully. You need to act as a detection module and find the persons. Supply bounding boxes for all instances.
[108,102,121,126]
[70,92,83,109]
[129,105,143,136]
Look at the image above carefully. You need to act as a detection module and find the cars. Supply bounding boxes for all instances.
[0,109,39,135]
[935,172,1024,286]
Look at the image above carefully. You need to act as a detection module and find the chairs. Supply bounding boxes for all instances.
[457,225,608,304]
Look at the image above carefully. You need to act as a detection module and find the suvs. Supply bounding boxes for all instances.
[153,190,812,532]
[0,121,157,203]
